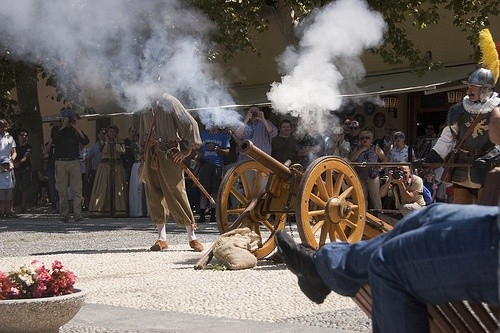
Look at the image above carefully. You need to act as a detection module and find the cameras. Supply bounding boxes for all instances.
[251,112,257,118]
[392,167,404,179]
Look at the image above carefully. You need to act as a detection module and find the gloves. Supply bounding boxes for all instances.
[423,152,443,164]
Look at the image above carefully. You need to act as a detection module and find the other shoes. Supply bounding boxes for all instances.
[74,218,83,222]
[59,216,69,221]
[197,217,207,223]
[209,215,216,222]
[189,239,204,252]
[0,212,16,218]
[150,240,168,251]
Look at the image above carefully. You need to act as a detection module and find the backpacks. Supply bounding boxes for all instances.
[419,186,434,205]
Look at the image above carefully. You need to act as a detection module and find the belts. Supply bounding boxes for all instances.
[56,157,84,161]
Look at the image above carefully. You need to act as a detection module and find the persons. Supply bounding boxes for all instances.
[0,69,500,252]
[275,203,500,333]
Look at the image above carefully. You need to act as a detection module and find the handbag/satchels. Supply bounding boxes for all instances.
[138,155,148,184]
[368,167,379,180]
[439,150,459,184]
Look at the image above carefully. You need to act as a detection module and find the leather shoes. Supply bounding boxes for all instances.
[274,228,332,304]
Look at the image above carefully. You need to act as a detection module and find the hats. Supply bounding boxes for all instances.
[394,131,405,139]
[359,130,374,140]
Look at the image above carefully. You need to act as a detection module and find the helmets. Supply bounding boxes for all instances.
[459,67,496,89]
